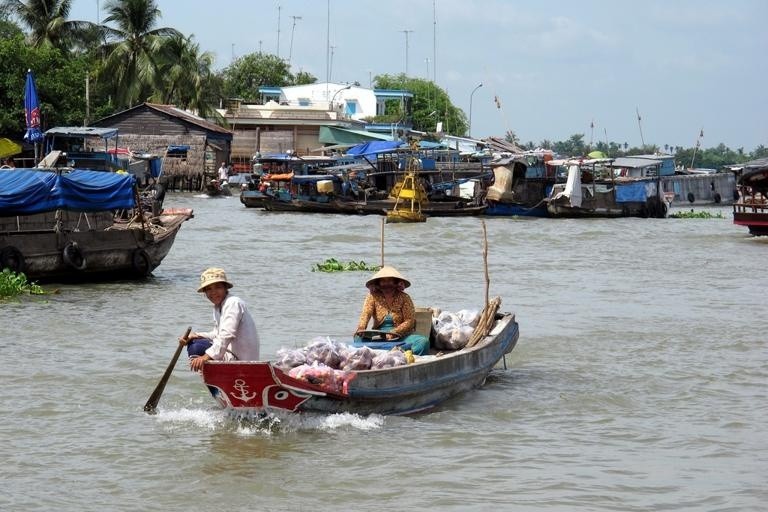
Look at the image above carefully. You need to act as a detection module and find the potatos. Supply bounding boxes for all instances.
[430,307,480,353]
[277,335,406,368]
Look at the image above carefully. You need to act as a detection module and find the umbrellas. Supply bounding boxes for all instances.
[0,136,23,159]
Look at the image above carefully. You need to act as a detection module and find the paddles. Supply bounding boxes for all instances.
[144,328,192,413]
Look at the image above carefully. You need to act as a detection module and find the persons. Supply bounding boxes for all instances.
[355,265,431,356]
[709,179,715,200]
[675,161,685,171]
[3,155,17,169]
[257,177,268,192]
[178,267,261,372]
[218,161,230,188]
[116,164,130,174]
[24,68,44,165]
[408,136,421,152]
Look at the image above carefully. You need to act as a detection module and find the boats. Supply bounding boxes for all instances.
[385,175,429,223]
[732,164,768,236]
[0,168,196,277]
[239,128,768,219]
[202,309,520,421]
[205,179,224,197]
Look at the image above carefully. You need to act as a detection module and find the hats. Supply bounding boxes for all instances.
[196,268,233,292]
[366,266,411,288]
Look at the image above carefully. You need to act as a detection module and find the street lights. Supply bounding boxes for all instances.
[469,82,483,136]
